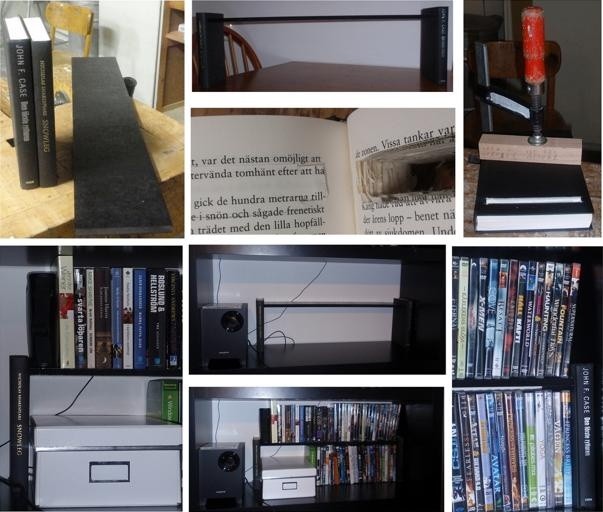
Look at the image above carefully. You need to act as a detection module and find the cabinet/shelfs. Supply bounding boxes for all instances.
[156,1,184,113]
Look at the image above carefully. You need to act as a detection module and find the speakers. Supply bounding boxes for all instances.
[198,442,245,506]
[200,303,248,369]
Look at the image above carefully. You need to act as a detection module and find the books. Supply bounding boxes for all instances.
[20,17,57,189]
[471,159,595,234]
[266,399,404,486]
[445,252,603,512]
[190,107,455,236]
[48,246,181,374]
[2,15,38,190]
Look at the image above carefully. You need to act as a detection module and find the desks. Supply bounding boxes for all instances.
[189,387,444,512]
[193,60,452,92]
[464,147,602,237]
[190,246,445,374]
[0,49,185,239]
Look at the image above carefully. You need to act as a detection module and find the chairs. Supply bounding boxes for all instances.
[45,1,94,61]
[486,40,562,137]
[192,17,262,84]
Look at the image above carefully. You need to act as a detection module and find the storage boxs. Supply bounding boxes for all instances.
[29,415,182,510]
[260,455,318,499]
